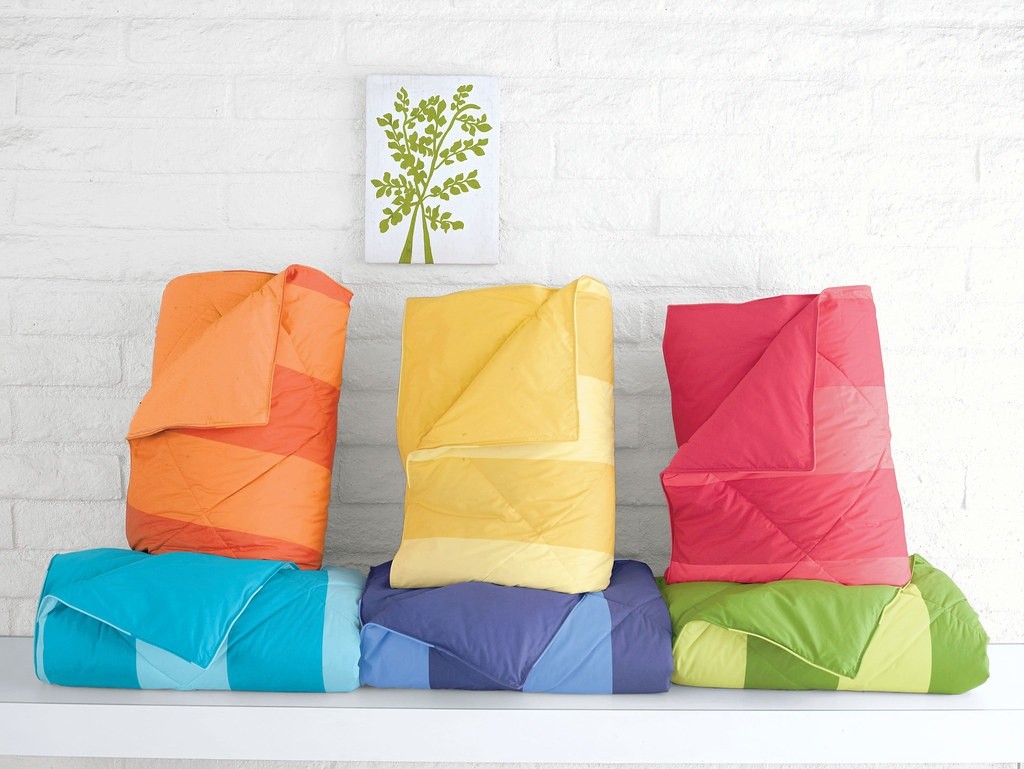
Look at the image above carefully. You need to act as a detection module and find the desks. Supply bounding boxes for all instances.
[0,637,1024,765]
